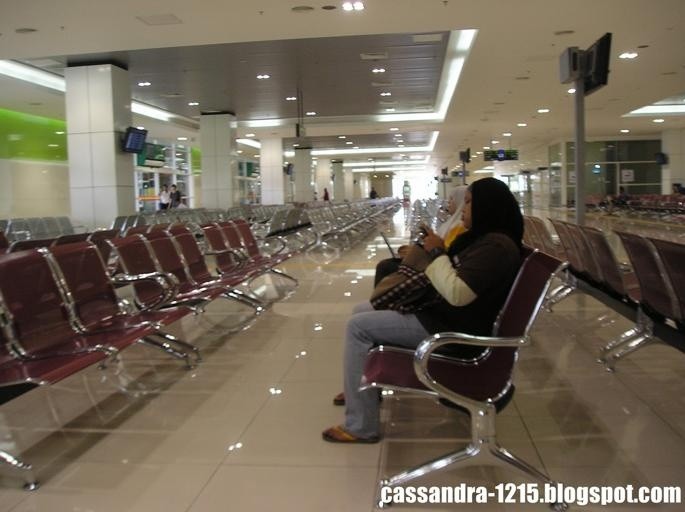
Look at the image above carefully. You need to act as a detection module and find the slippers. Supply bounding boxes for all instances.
[332,396,384,406]
[321,425,382,445]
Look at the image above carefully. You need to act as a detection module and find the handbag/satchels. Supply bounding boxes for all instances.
[368,242,463,316]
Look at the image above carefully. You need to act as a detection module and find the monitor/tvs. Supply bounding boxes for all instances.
[464,148,471,163]
[287,163,294,174]
[585,31,611,96]
[123,126,147,152]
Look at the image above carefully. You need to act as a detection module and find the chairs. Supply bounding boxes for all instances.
[0,192,684,512]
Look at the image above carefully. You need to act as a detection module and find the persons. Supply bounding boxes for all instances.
[618,186,627,200]
[159,184,187,209]
[673,182,685,195]
